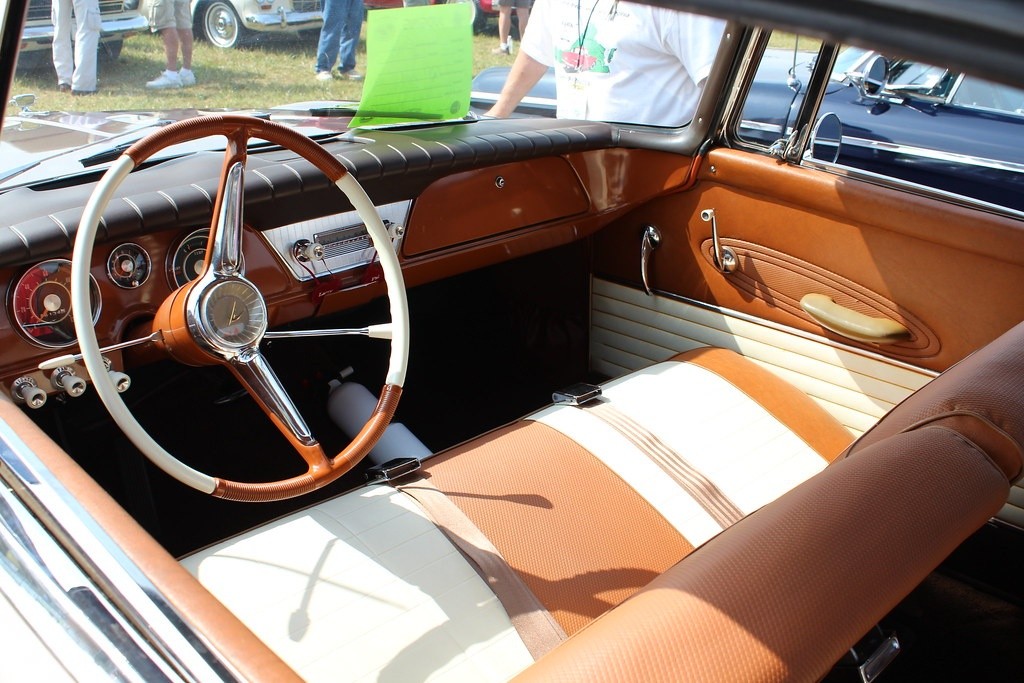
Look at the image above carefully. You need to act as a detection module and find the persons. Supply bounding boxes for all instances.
[145,0,199,90]
[314,0,365,82]
[51,0,102,96]
[492,0,535,57]
[482,0,727,127]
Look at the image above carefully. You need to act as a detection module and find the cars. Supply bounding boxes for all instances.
[0,1,150,72]
[0,0,1024,681]
[469,39,1022,217]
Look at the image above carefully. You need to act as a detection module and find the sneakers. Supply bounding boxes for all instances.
[339,69,362,80]
[315,71,333,81]
[145,72,183,89]
[492,45,509,55]
[178,71,196,85]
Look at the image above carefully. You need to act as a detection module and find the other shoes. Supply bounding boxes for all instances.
[70,90,99,96]
[60,84,71,92]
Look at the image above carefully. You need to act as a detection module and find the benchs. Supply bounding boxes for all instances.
[177,320,1024,683]
